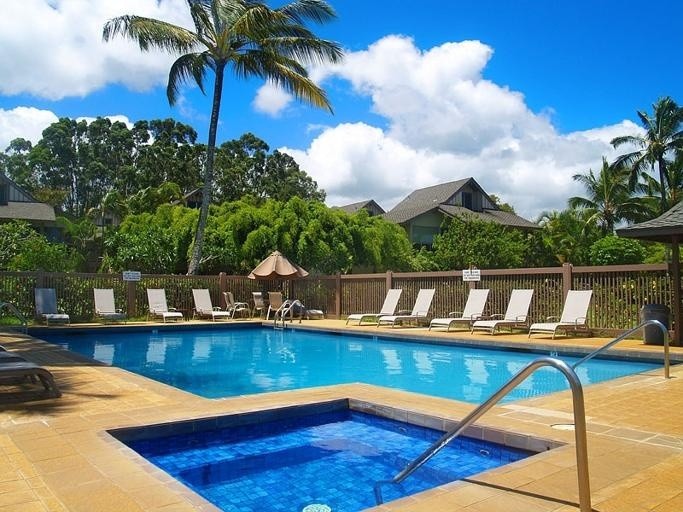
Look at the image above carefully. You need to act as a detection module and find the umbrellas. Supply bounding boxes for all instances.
[247,251,309,291]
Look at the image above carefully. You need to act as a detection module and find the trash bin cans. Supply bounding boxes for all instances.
[640,304,669,345]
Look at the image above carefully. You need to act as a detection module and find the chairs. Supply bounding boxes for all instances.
[192,289,324,322]
[34,287,70,328]
[147,288,184,322]
[93,288,128,326]
[0,360,63,400]
[429,289,491,333]
[471,288,534,336]
[345,288,403,326]
[377,287,437,329]
[535,289,593,340]
